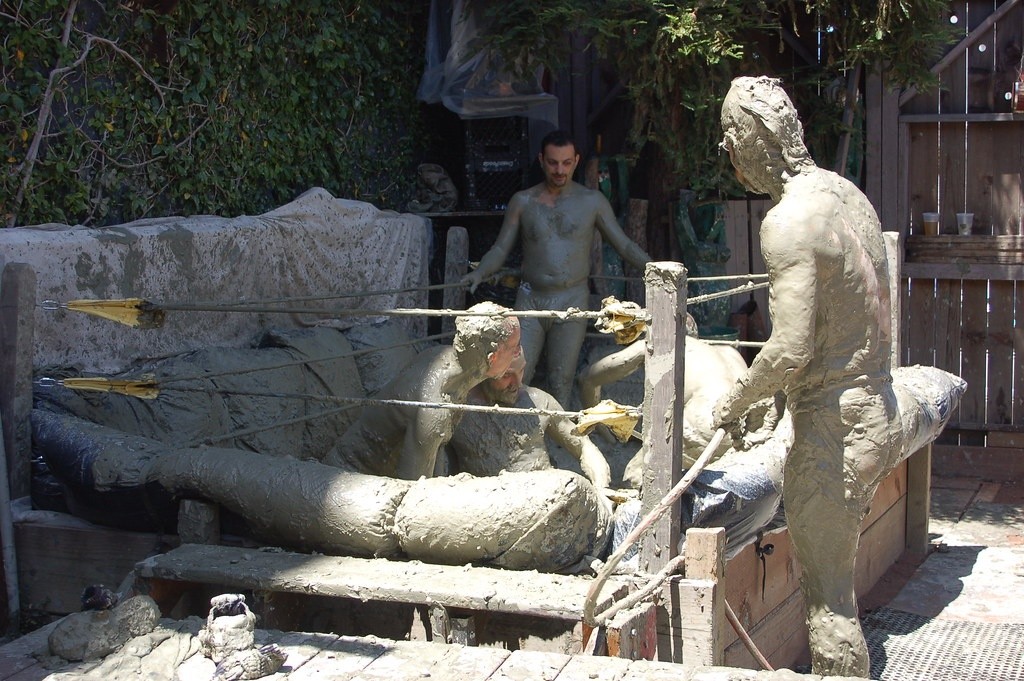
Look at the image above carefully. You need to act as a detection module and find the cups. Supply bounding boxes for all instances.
[922,212,938,238]
[956,213,974,237]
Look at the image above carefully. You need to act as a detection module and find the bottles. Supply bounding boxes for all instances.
[461,164,479,204]
[1011,52,1024,114]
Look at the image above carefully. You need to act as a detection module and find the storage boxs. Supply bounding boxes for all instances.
[465,116,528,211]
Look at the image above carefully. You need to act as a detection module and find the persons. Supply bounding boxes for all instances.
[579,313,748,489]
[710,76,902,678]
[316,301,520,480]
[433,345,611,490]
[456,130,652,410]
